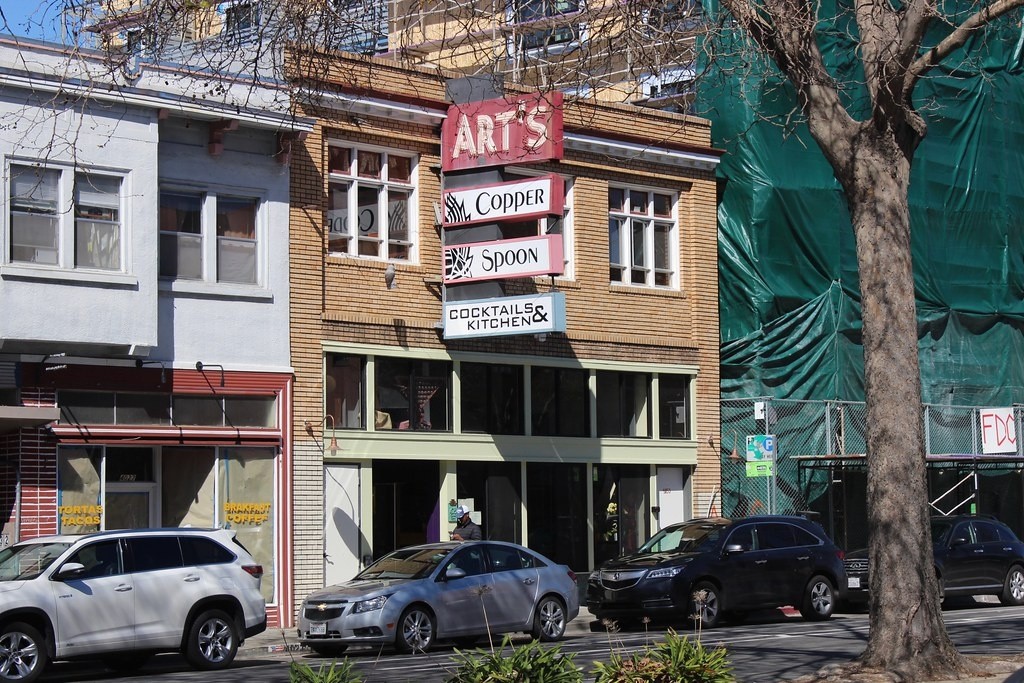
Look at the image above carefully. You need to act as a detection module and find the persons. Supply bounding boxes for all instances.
[450,505,482,542]
[57,541,118,580]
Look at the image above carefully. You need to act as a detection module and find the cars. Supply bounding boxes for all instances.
[844,513,1023,607]
[586,515,849,628]
[295,540,580,657]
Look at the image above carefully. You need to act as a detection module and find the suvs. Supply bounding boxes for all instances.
[1,526,267,682]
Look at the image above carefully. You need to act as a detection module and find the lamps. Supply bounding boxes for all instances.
[136,358,166,384]
[196,361,224,387]
[306,414,343,456]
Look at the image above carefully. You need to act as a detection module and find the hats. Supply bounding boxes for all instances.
[457,504,469,519]
